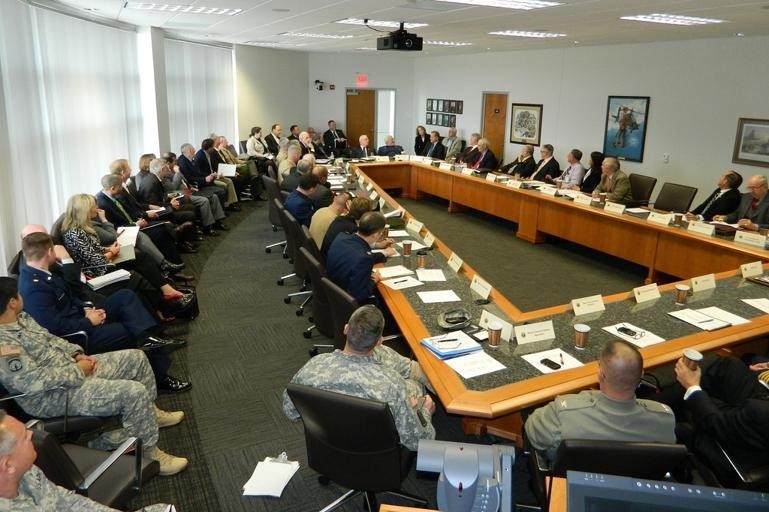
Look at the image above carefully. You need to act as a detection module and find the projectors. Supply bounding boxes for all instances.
[377,38,422,50]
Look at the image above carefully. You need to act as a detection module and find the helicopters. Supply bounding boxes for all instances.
[610,102,645,133]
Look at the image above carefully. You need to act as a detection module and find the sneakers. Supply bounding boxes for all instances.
[225,202,244,213]
[191,221,232,242]
[150,401,187,430]
[253,192,269,202]
[143,445,190,478]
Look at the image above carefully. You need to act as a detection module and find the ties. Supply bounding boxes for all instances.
[472,153,483,170]
[558,165,572,181]
[700,192,719,215]
[362,146,368,157]
[333,131,339,139]
[113,198,136,226]
[751,196,759,211]
[427,143,435,157]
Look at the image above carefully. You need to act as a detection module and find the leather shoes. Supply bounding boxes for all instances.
[156,314,178,325]
[155,373,194,396]
[134,334,167,351]
[159,334,189,353]
[161,290,186,305]
[159,260,196,283]
[178,236,201,255]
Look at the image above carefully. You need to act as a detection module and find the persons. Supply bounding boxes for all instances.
[414,126,499,171]
[499,144,560,182]
[545,148,632,204]
[17,131,263,396]
[377,135,403,156]
[1,408,170,512]
[248,120,396,306]
[685,170,769,232]
[281,304,438,453]
[523,340,769,468]
[0,277,188,476]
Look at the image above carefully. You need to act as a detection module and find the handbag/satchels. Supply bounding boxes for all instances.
[163,282,200,322]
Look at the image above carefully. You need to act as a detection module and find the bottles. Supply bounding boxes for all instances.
[331,152,335,159]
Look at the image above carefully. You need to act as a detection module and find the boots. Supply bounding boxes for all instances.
[408,359,438,397]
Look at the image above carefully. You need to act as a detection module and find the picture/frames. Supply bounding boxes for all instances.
[425,99,463,128]
[732,117,769,168]
[602,95,650,163]
[510,103,543,146]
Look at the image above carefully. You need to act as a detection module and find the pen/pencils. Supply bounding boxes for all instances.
[438,339,457,342]
[394,280,407,284]
[117,229,125,237]
[698,319,713,323]
[560,353,563,364]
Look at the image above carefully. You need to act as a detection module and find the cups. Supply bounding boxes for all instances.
[515,173,520,179]
[402,241,412,257]
[488,324,502,347]
[382,224,390,237]
[401,151,406,155]
[683,350,704,370]
[574,323,591,351]
[673,284,691,305]
[760,228,768,235]
[451,158,456,164]
[556,180,563,189]
[335,161,352,184]
[673,213,683,227]
[599,192,607,205]
[417,251,428,270]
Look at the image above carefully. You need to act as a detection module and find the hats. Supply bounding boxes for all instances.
[435,306,474,332]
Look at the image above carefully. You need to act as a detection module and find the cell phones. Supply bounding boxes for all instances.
[447,317,466,323]
[617,327,636,336]
[541,359,561,370]
